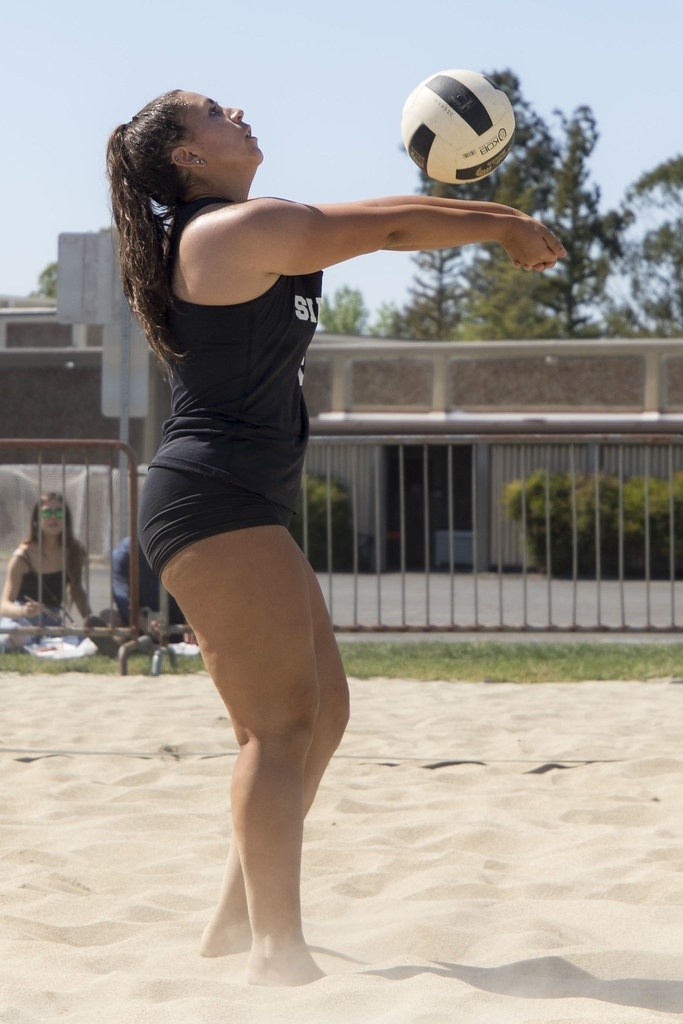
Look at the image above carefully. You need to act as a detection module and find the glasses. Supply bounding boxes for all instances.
[38,507,63,518]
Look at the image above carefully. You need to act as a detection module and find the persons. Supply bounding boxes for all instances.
[84,526,199,660]
[0,490,98,657]
[104,89,569,994]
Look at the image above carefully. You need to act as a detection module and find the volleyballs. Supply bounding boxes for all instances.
[399,67,517,186]
